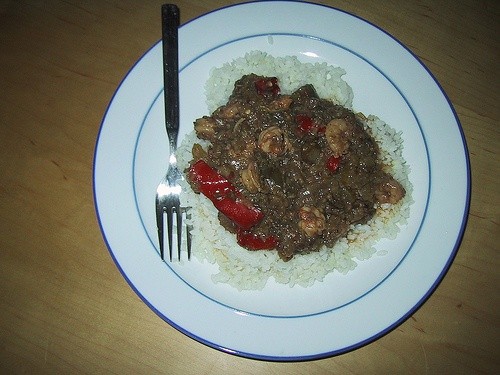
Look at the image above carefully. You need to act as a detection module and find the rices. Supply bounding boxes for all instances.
[175,34,416,286]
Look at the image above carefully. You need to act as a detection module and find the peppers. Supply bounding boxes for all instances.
[236,227,279,251]
[188,161,264,231]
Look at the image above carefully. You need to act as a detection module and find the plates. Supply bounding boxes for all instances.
[95,1,473,362]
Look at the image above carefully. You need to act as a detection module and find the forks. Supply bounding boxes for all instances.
[152,2,194,263]
